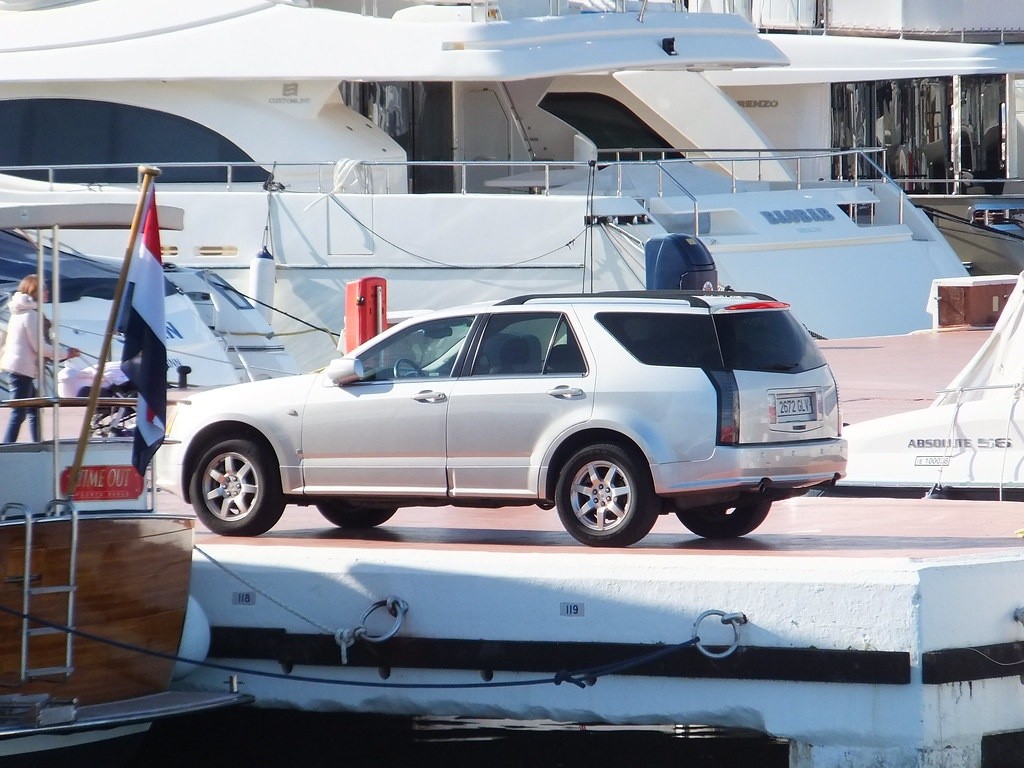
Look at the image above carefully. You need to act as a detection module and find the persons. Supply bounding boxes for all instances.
[0,274,82,444]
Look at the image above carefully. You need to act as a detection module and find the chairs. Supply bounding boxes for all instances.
[549,344,577,373]
[499,335,541,374]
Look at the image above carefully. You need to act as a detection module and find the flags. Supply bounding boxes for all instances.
[113,185,171,478]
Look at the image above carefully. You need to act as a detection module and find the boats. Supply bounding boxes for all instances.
[1,168,244,768]
[0,0,975,394]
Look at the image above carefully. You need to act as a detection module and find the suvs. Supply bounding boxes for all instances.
[150,287,851,552]
